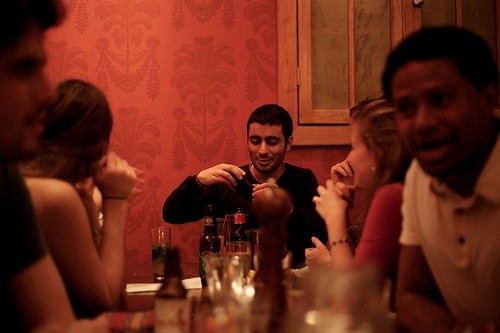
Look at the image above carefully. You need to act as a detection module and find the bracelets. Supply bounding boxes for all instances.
[102,195,129,202]
[328,237,351,247]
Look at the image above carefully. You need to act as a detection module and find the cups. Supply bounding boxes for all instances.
[197,214,384,333]
[150,226,171,282]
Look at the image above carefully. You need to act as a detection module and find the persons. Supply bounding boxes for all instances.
[303,25,500,333]
[162,104,327,269]
[0,0,143,333]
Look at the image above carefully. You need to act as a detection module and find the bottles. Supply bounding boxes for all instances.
[196,253,251,333]
[154,247,191,333]
[231,207,248,241]
[199,204,221,288]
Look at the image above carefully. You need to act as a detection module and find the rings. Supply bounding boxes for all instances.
[313,197,318,200]
